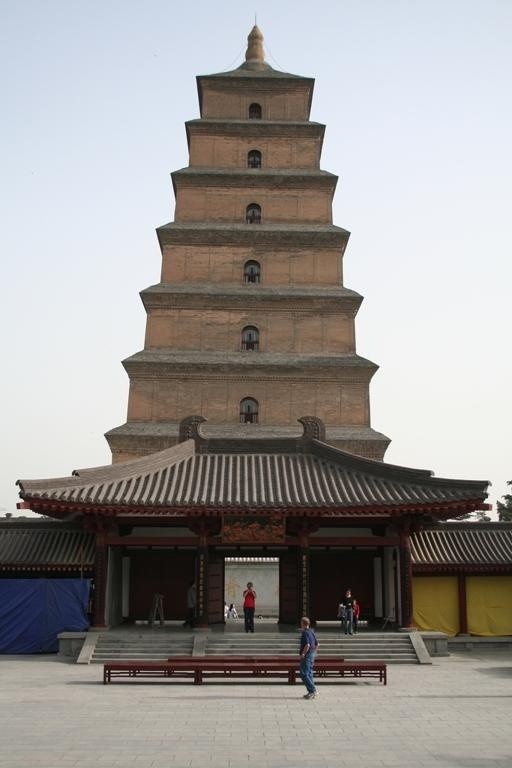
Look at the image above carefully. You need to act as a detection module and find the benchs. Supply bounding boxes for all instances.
[100,656,387,684]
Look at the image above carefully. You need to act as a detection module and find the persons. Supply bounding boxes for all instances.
[224,601,238,619]
[340,588,360,634]
[181,580,198,630]
[243,582,257,632]
[299,616,319,699]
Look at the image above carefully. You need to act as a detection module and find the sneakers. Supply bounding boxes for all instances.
[305,690,319,699]
